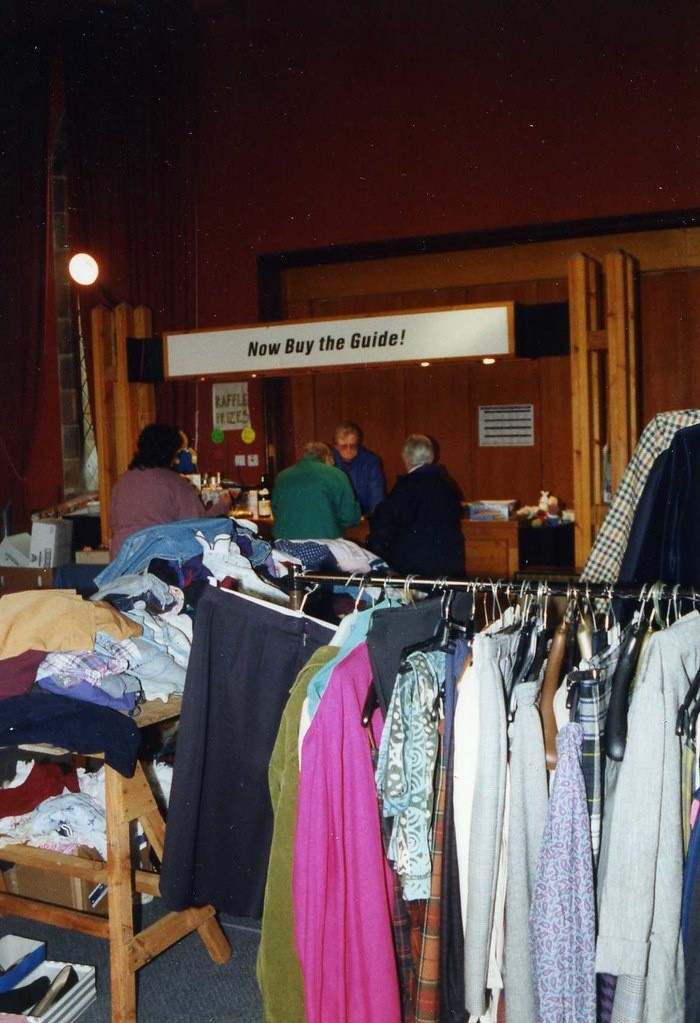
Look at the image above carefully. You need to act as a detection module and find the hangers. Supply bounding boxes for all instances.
[206,569,700,768]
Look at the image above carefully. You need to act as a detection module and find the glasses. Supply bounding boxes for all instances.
[337,443,357,451]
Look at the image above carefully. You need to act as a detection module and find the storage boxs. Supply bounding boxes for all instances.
[0,960,96,1023]
[0,934,46,993]
[4,847,150,912]
[0,532,31,568]
[29,518,73,567]
[469,500,517,521]
[76,551,110,565]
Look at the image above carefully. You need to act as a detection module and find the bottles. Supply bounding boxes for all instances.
[257,473,271,517]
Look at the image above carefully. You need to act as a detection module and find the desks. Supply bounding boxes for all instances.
[0,695,232,1023]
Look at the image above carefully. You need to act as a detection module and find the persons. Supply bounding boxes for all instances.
[269,442,361,542]
[328,420,385,513]
[359,433,467,582]
[106,420,232,568]
